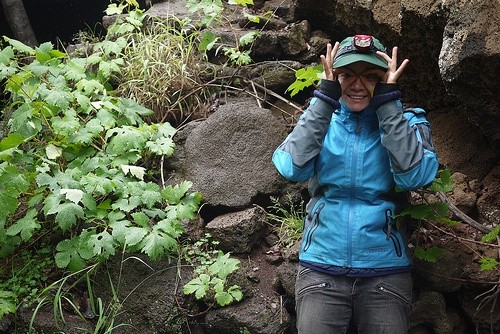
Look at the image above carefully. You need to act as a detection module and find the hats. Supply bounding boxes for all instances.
[332,35,388,68]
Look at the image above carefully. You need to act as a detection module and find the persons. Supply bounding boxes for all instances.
[271,34,438,334]
